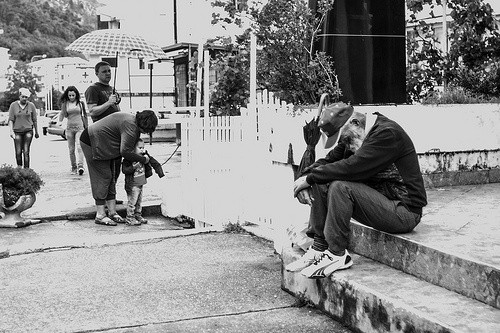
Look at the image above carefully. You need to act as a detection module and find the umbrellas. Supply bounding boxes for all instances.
[63,28,167,105]
[295,93,331,180]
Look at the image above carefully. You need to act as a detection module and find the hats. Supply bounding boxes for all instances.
[317,101,355,148]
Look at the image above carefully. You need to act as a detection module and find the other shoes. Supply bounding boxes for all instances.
[78,167,84,175]
[108,213,125,223]
[95,216,117,226]
[126,217,141,225]
[71,169,77,174]
[135,215,147,223]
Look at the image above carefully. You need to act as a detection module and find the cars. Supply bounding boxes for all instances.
[41,110,62,135]
[46,104,93,140]
[0,111,9,126]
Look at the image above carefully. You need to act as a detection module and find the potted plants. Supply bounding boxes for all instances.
[0,164,45,227]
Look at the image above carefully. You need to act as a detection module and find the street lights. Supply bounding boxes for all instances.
[30,53,47,77]
[127,56,145,110]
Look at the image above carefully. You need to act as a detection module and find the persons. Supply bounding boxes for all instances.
[121,138,165,227]
[284,101,427,279]
[58,86,89,175]
[84,61,122,205]
[7,88,39,169]
[79,110,158,226]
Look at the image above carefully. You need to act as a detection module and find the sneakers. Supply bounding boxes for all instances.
[301,249,353,278]
[286,245,324,272]
